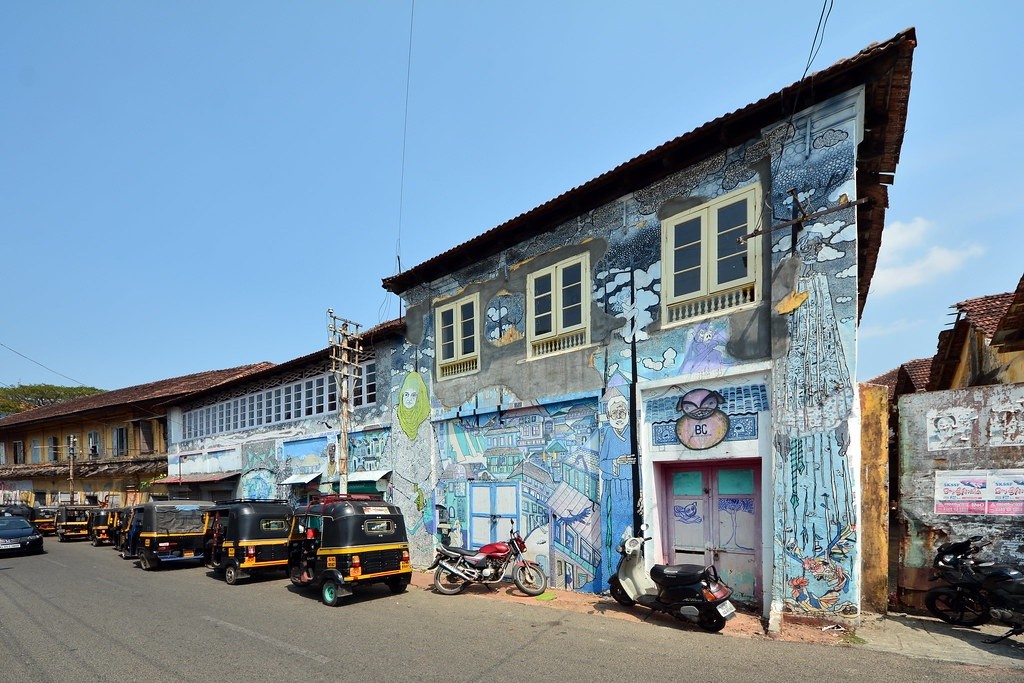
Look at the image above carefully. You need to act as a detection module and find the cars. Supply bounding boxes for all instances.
[0,515,44,556]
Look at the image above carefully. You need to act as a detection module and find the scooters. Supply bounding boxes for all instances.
[607,523,737,632]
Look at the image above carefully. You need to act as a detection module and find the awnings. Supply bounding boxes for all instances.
[276,472,323,484]
[322,469,392,481]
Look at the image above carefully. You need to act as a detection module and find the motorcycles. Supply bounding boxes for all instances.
[924,535,1024,644]
[29,504,137,559]
[127,501,217,571]
[286,494,414,607]
[428,517,547,596]
[200,498,301,585]
